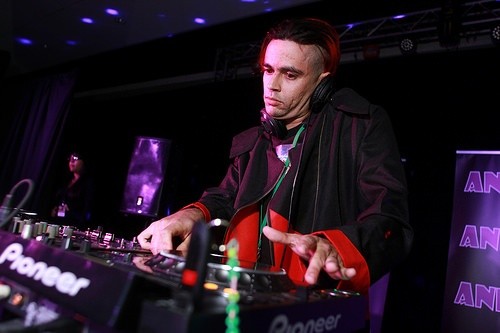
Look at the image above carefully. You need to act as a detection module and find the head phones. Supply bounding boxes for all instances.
[259,75,335,139]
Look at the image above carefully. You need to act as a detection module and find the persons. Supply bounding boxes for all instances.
[137,18,414,333]
[49,148,103,232]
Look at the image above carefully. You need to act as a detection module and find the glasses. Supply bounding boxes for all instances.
[69,157,79,161]
